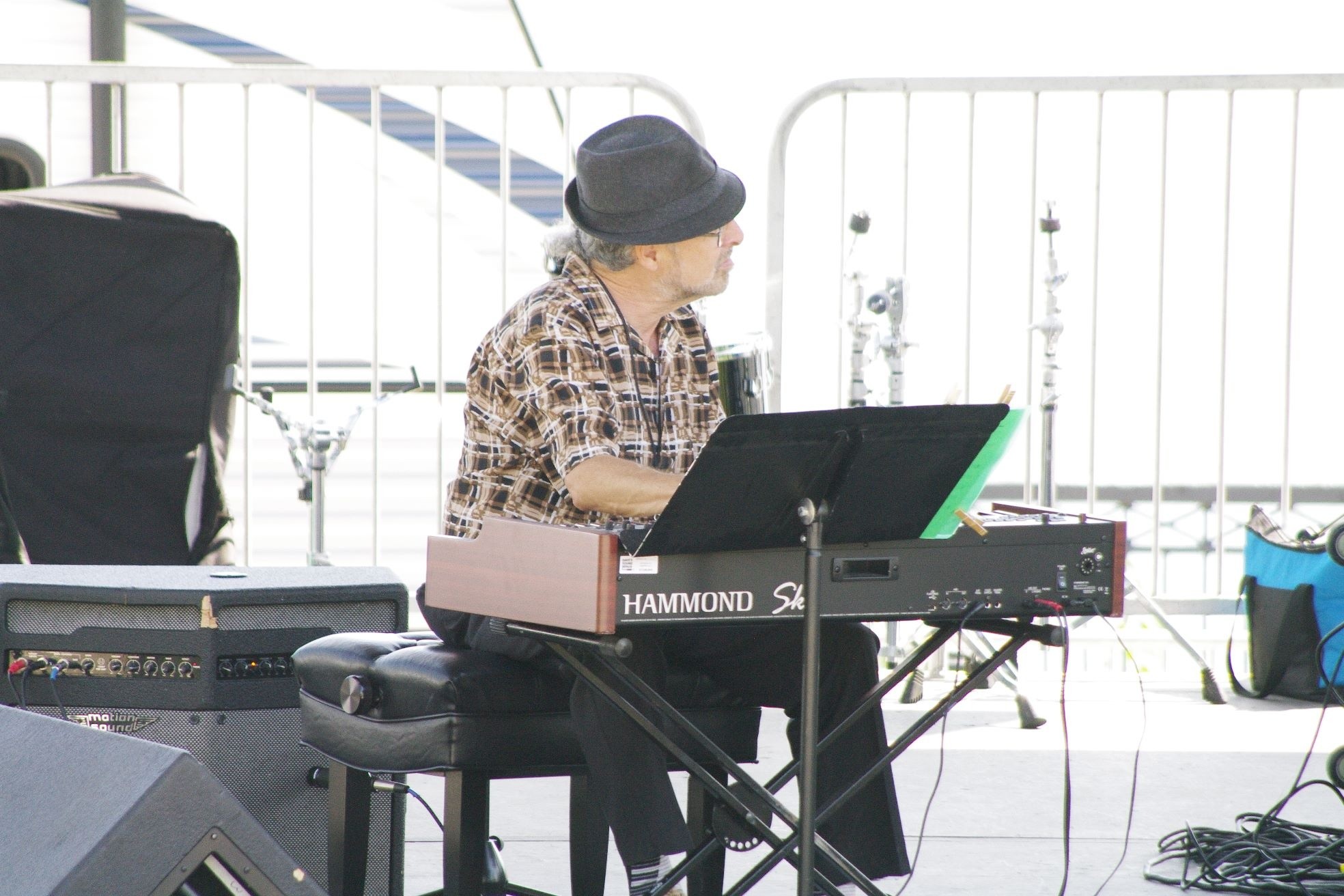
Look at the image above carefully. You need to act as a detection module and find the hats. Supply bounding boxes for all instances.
[564,115,745,245]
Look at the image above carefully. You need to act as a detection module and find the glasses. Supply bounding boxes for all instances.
[701,228,724,248]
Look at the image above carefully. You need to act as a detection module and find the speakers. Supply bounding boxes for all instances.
[0,562,407,896]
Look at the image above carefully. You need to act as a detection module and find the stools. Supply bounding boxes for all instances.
[289,625,762,895]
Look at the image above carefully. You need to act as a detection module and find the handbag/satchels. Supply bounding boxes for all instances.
[1226,499,1343,705]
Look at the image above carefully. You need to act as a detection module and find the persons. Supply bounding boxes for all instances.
[415,122,912,896]
